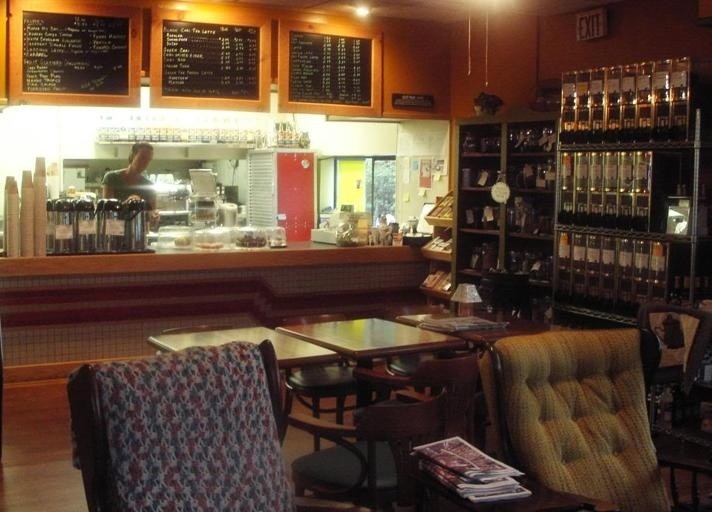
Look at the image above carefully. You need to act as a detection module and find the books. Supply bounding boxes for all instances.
[407,433,536,505]
[415,316,510,333]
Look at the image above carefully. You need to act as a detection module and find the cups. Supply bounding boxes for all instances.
[5,156,49,258]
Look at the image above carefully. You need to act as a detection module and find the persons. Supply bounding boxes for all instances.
[99,138,159,217]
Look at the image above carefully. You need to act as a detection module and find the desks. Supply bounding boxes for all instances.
[410,471,584,512]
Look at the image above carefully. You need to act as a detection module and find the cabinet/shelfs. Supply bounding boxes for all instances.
[245,147,316,242]
[554,55,712,327]
[451,111,559,320]
[419,189,452,305]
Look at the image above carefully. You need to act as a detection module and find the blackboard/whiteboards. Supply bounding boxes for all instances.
[278,20,382,116]
[6,1,143,106]
[149,8,272,112]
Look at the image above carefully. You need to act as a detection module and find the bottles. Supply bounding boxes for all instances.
[507,211,515,232]
[96,115,255,143]
[277,121,300,148]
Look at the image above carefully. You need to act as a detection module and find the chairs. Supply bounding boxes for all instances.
[279,311,365,454]
[65,338,370,512]
[476,326,712,512]
[349,346,480,447]
[292,388,453,512]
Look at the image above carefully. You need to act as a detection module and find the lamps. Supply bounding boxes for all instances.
[450,283,483,318]
